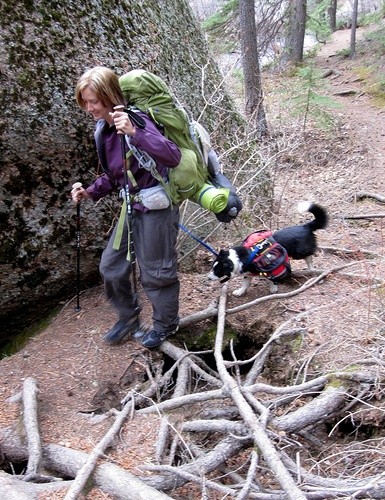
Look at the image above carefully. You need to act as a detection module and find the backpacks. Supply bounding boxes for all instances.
[119,69,243,227]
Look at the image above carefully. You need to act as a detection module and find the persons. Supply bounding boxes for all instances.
[70,65,182,348]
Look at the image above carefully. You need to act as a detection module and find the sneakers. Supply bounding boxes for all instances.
[141,322,178,347]
[103,317,138,346]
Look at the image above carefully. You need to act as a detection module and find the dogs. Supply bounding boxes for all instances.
[208,201,329,297]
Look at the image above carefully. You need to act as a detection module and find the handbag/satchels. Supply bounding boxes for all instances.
[134,179,172,212]
